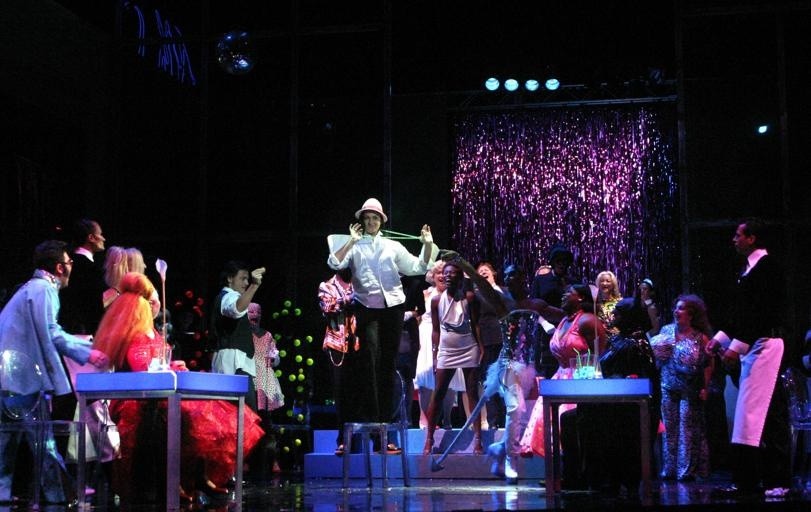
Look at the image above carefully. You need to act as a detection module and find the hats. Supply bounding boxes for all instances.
[354,197,390,223]
[550,246,576,265]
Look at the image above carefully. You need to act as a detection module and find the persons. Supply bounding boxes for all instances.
[316,197,811,497]
[0,216,289,512]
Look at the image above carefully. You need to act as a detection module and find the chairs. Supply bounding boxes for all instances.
[342,369,411,489]
[0,348,86,511]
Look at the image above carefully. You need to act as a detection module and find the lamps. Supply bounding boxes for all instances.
[485,69,560,91]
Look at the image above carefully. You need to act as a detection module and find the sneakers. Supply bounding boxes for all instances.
[484,442,506,479]
[505,454,521,481]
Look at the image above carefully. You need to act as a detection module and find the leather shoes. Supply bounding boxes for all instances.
[472,435,485,456]
[423,439,435,457]
[374,442,404,456]
[334,440,358,456]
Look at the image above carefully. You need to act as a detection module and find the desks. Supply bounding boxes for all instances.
[75,371,248,512]
[538,379,654,493]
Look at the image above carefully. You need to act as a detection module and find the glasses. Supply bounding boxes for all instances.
[59,258,76,266]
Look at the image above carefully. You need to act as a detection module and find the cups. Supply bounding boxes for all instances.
[570,358,588,379]
[143,343,172,373]
[582,354,598,379]
